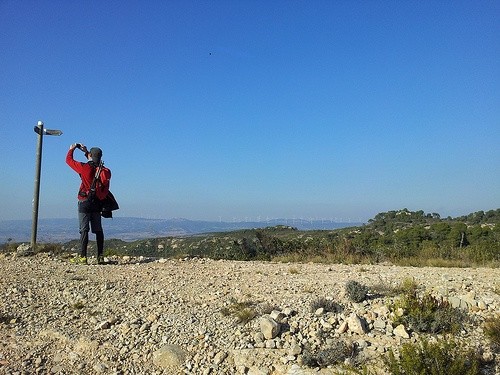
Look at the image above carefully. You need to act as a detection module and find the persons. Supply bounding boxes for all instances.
[66,143,105,265]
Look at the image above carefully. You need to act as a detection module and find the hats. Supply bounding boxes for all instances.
[90,147,102,163]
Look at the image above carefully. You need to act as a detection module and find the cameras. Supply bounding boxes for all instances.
[76,144,81,148]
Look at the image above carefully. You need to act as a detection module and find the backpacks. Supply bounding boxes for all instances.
[83,161,111,203]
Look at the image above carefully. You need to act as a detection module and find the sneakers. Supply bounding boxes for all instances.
[92,255,104,264]
[68,255,87,265]
